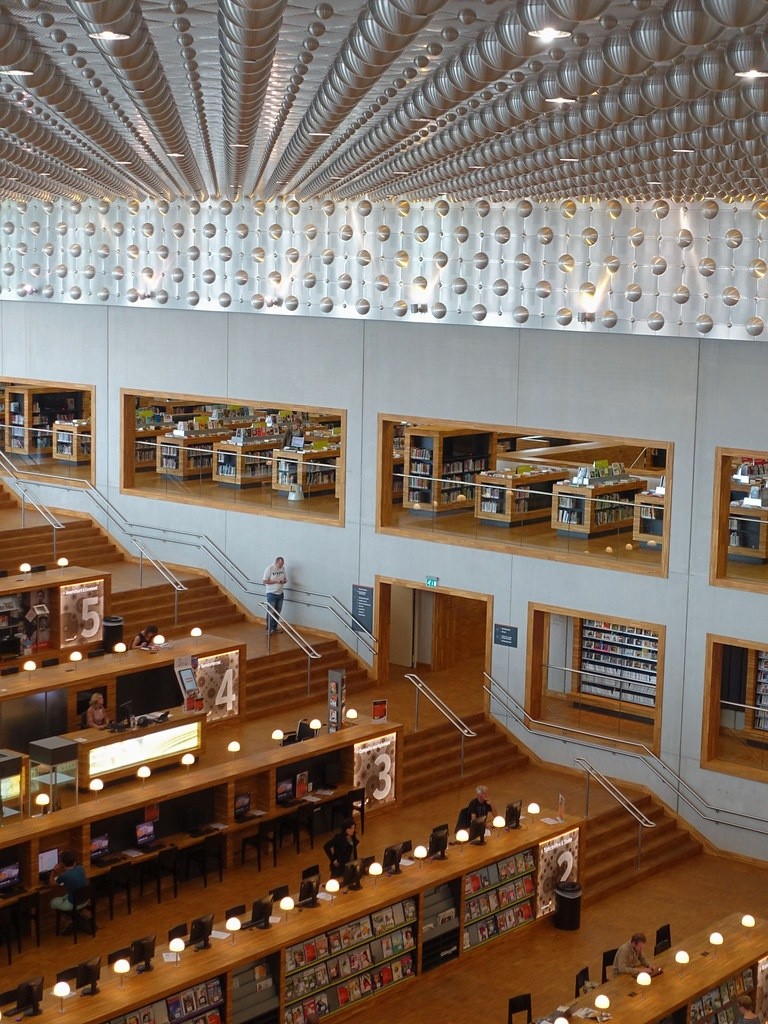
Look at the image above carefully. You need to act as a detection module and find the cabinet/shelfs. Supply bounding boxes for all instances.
[537,912,767,1023]
[739,649,768,742]
[0,812,588,1022]
[0,720,404,926]
[570,616,660,709]
[0,566,112,672]
[0,381,767,567]
[0,633,246,753]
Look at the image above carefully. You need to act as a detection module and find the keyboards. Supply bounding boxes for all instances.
[191,829,214,838]
[294,898,318,907]
[96,857,120,867]
[239,919,264,929]
[235,815,255,824]
[0,886,25,899]
[282,799,306,808]
[184,939,200,947]
[339,881,353,888]
[3,1004,32,1017]
[426,851,438,858]
[142,844,166,854]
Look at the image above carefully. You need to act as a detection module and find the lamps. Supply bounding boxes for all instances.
[53,878,340,1013]
[555,914,756,1023]
[20,556,359,808]
[369,801,540,879]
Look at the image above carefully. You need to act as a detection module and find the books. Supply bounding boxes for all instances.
[392,425,665,525]
[111,898,414,1024]
[755,650,768,729]
[329,676,346,733]
[0,595,20,627]
[463,849,535,949]
[0,382,91,455]
[134,399,341,485]
[580,619,658,705]
[690,968,754,1024]
[728,462,768,546]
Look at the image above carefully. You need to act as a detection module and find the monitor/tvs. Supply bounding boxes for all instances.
[116,700,134,727]
[276,775,296,805]
[0,792,523,1017]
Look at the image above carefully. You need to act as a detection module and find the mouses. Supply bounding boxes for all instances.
[258,815,262,818]
[409,856,413,860]
[448,842,454,845]
[121,857,126,860]
[214,828,219,830]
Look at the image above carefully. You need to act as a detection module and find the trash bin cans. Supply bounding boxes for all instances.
[555,881,582,930]
[102,616,124,654]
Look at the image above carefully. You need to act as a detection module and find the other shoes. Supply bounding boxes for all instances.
[266,629,272,636]
[272,629,283,633]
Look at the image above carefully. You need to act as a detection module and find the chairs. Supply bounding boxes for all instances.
[600,949,618,985]
[576,966,589,998]
[508,993,532,1024]
[654,922,672,956]
[0,789,368,964]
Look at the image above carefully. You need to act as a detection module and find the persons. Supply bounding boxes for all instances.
[132,625,158,649]
[469,785,498,828]
[87,692,109,730]
[324,818,360,877]
[297,774,308,798]
[263,556,287,636]
[404,931,414,949]
[49,852,91,934]
[12,591,49,642]
[281,418,303,449]
[613,933,654,976]
[734,995,761,1024]
